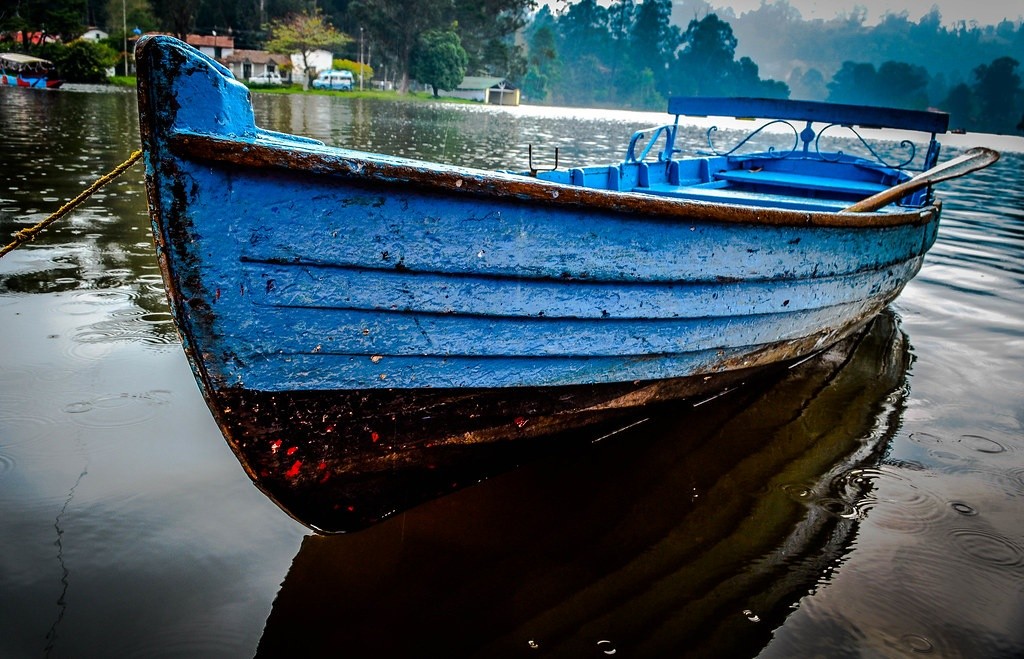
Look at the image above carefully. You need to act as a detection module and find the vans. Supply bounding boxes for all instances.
[313,71,355,91]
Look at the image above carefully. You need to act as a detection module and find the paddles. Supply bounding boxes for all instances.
[836,145,1000,213]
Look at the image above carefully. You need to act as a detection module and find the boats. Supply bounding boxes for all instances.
[0,52,68,89]
[254,308,917,659]
[132,32,955,537]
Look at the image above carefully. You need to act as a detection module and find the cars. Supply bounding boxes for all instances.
[248,72,283,85]
